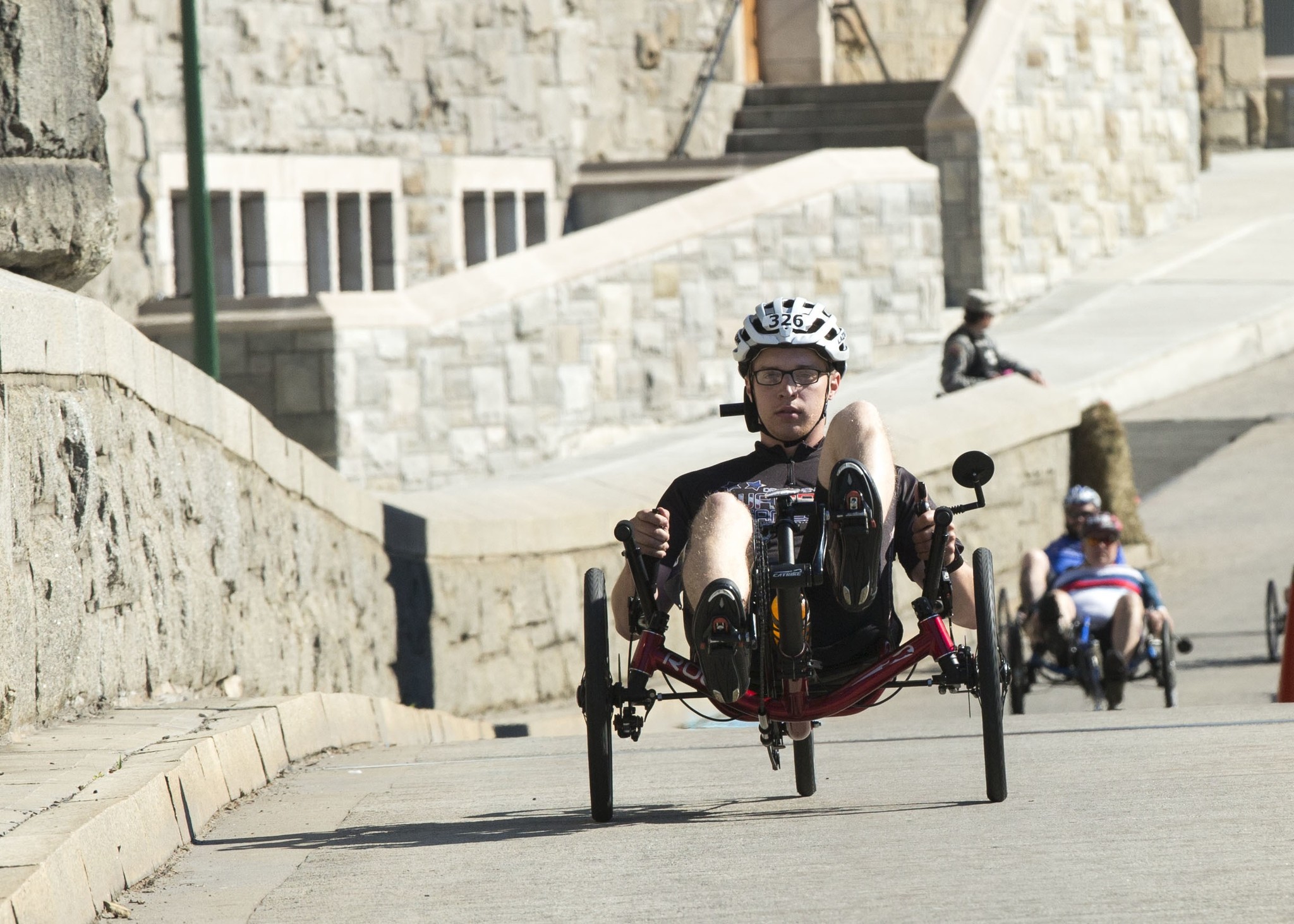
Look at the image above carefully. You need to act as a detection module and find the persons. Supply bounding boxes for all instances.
[1021,484,1129,617]
[609,297,978,720]
[1022,514,1177,702]
[942,287,1046,398]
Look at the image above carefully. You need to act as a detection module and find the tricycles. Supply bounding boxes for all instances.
[573,448,1014,825]
[997,584,1196,715]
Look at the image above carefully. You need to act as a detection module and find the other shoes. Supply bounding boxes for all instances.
[691,577,750,705]
[1103,651,1127,707]
[1055,628,1077,680]
[825,458,883,613]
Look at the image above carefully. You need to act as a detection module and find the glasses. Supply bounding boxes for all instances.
[751,368,832,386]
[1084,530,1120,545]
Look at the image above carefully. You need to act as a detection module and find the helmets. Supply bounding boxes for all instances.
[732,297,850,380]
[1063,485,1101,508]
[1078,511,1126,532]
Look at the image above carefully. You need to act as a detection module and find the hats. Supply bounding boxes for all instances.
[964,288,1003,316]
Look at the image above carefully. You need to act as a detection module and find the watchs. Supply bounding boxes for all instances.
[943,535,967,576]
